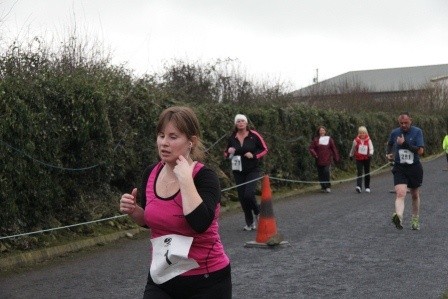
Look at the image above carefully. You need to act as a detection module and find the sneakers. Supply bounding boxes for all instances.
[356,186,361,192]
[321,189,326,193]
[326,188,330,192]
[243,222,257,231]
[365,188,370,192]
[393,214,403,230]
[411,216,421,230]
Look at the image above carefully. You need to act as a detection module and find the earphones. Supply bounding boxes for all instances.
[190,141,192,147]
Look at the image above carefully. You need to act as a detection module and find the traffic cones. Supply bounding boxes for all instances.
[244,176,289,250]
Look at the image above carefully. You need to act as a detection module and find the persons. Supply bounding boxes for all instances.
[223,113,269,232]
[348,126,374,194]
[309,126,339,193]
[118,107,233,299]
[442,131,448,171]
[387,111,425,231]
